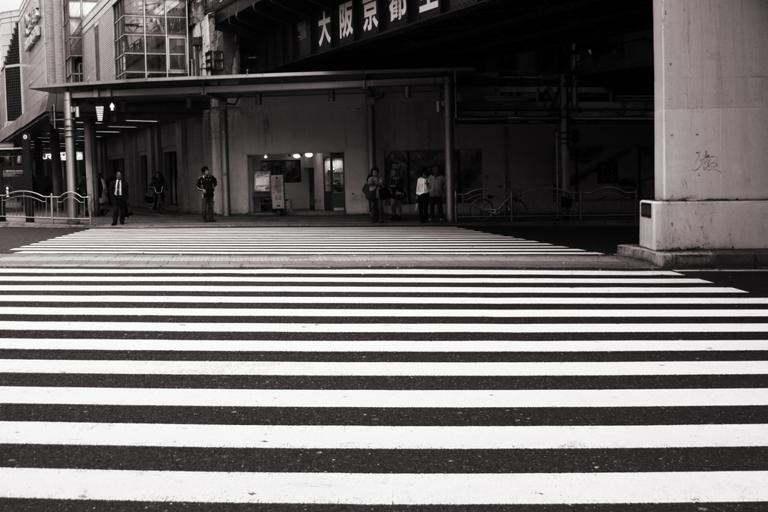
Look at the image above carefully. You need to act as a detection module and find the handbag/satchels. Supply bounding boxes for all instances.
[394,191,406,200]
[415,203,418,211]
[379,188,391,200]
[366,190,376,200]
[362,184,369,192]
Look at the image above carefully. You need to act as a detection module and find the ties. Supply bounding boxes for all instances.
[117,180,119,196]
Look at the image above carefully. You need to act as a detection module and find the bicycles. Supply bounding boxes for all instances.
[469,183,527,222]
[149,184,166,213]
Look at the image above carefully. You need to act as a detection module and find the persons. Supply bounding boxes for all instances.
[366,167,446,224]
[148,171,168,211]
[111,171,129,226]
[194,166,218,223]
